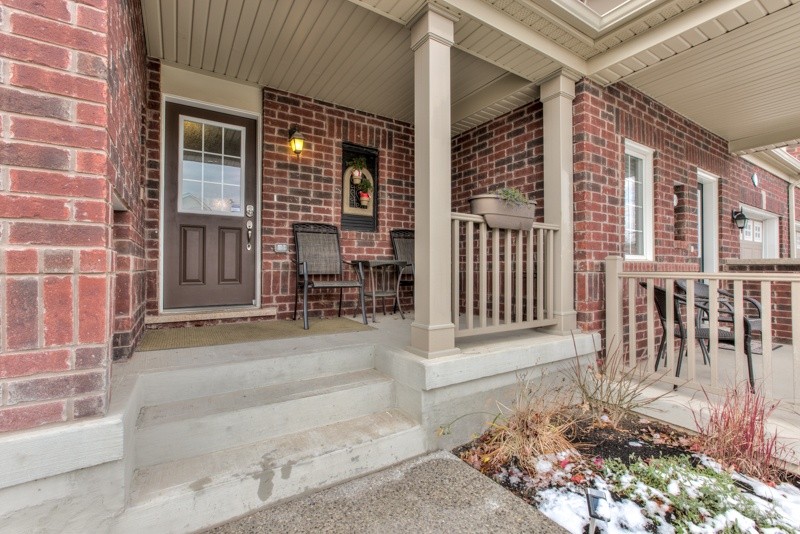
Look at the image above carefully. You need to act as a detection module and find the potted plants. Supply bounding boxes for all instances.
[356,178,373,205]
[346,156,368,185]
[468,187,538,231]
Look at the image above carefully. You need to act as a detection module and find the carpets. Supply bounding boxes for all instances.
[133,316,379,352]
[718,338,783,356]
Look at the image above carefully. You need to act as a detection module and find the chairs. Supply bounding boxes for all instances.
[390,229,415,313]
[292,223,368,330]
[639,277,763,394]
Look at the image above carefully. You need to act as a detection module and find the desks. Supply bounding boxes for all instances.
[343,259,412,323]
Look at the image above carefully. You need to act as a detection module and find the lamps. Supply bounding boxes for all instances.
[289,131,306,159]
[731,206,747,229]
